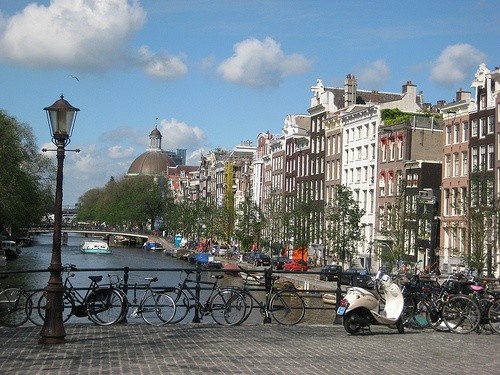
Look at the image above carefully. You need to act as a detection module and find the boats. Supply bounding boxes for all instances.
[80,238,112,254]
[142,239,166,252]
[0,237,31,278]
[162,247,223,270]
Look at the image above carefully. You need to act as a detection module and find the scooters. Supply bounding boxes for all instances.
[336,273,407,335]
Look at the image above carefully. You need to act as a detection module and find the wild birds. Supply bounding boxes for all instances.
[72,76,79,81]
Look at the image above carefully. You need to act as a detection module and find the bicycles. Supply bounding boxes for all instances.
[154,265,307,327]
[376,259,500,334]
[24,262,176,326]
[0,274,33,327]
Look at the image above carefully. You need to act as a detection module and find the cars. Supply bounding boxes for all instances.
[184,238,228,256]
[242,251,308,272]
[319,265,371,288]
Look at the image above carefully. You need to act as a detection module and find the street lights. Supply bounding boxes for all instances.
[36,92,81,345]
